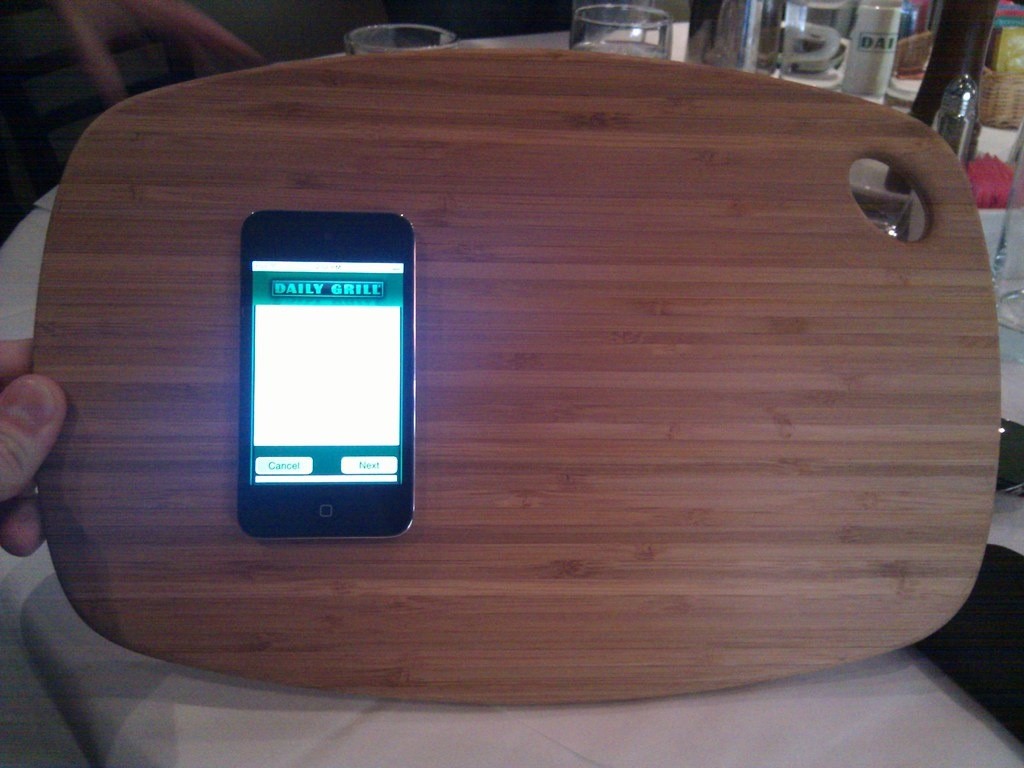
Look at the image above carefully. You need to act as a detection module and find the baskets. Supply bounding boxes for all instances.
[976,65,1024,130]
[895,32,934,79]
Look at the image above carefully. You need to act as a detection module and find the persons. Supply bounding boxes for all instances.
[0,337,68,555]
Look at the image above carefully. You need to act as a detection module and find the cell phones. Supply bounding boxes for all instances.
[236,212,415,540]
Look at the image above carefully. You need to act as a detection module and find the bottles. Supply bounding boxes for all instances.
[783,0,999,170]
[992,116,1024,333]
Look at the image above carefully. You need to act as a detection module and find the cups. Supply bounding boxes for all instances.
[345,22,457,56]
[570,0,674,60]
[683,0,785,75]
